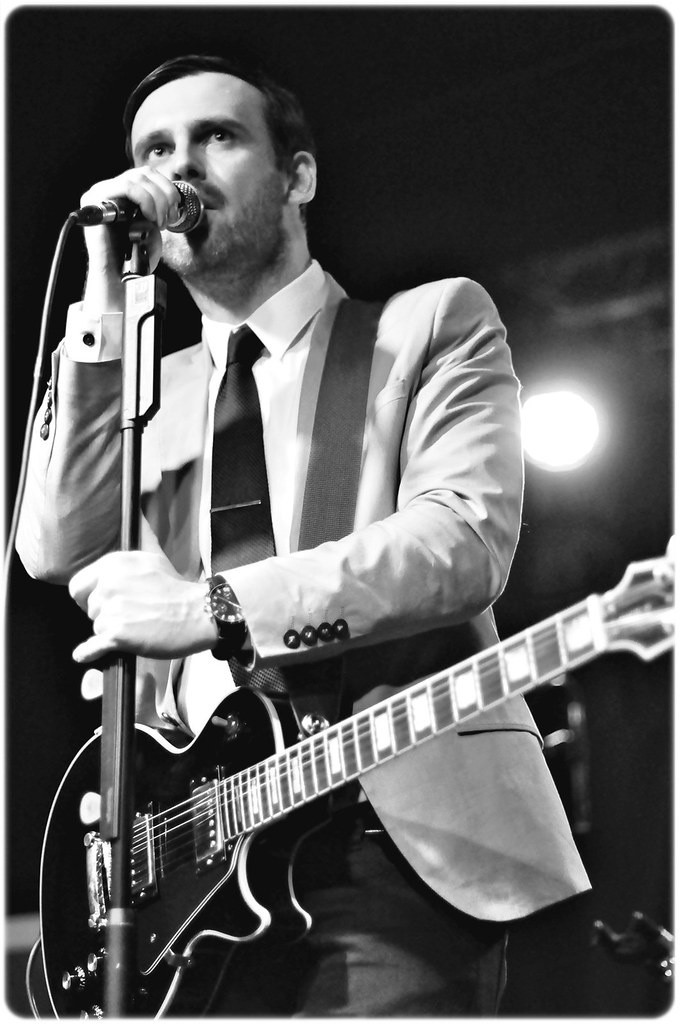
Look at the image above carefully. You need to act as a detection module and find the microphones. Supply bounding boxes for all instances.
[69,181,203,232]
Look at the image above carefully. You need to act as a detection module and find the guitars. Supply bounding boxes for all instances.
[39,538,675,1018]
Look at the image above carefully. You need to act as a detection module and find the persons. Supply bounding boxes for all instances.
[13,51,597,1017]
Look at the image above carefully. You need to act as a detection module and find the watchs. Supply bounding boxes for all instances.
[203,574,250,661]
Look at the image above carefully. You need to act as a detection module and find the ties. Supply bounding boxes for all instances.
[210,324,291,694]
